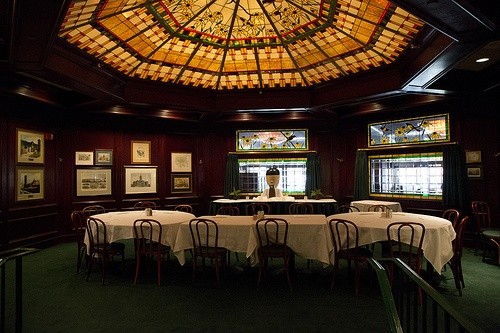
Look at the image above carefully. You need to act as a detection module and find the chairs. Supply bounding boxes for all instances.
[175,203,314,292]
[471,200,500,266]
[328,205,470,306]
[70,201,170,287]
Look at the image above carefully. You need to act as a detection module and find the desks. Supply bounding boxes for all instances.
[327,212,457,293]
[349,200,402,213]
[84,211,195,278]
[212,198,337,215]
[173,214,334,282]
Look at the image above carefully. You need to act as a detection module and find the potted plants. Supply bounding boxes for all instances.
[310,189,324,199]
[230,187,240,200]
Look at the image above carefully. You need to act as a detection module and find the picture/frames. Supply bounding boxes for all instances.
[74,149,94,167]
[123,164,159,195]
[463,149,483,163]
[130,140,152,165]
[15,127,45,166]
[15,167,45,202]
[464,164,485,181]
[94,149,114,167]
[171,174,193,194]
[170,151,193,173]
[75,166,113,197]
